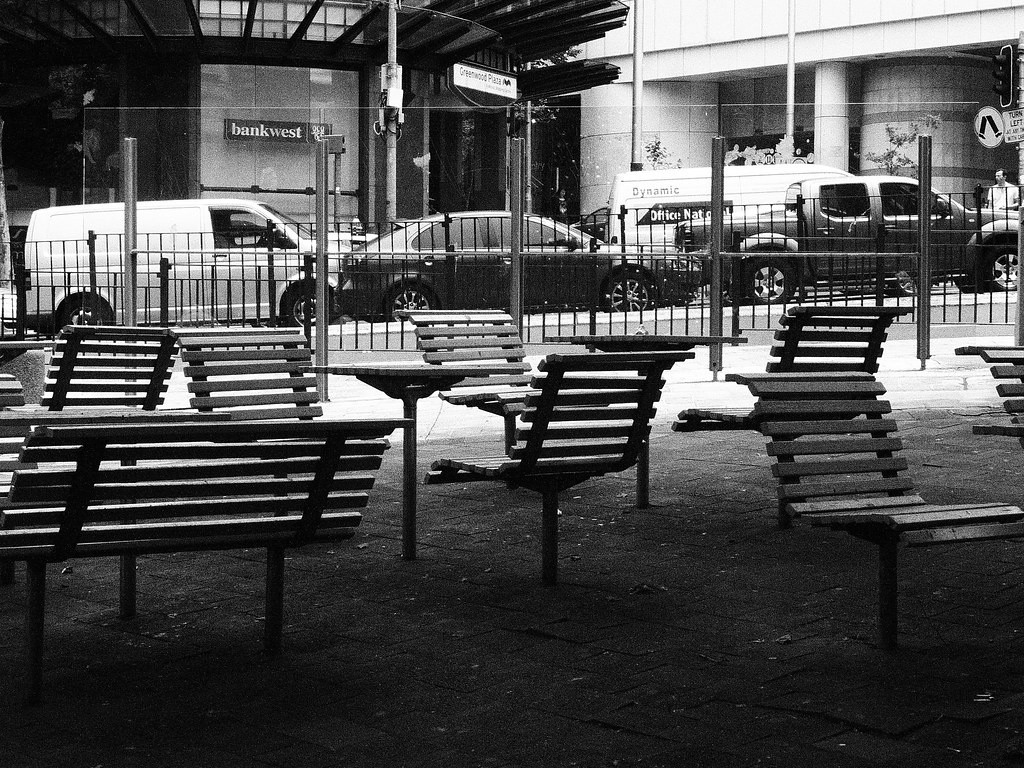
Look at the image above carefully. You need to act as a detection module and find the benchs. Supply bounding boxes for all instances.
[0,306,1024,663]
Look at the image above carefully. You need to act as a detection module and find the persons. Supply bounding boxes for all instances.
[987,167,1020,211]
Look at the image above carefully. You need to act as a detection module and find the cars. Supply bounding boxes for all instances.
[336,209,699,313]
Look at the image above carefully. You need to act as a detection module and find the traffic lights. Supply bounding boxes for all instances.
[990,42,1019,108]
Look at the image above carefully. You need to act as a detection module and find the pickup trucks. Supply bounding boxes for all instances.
[674,173,1024,305]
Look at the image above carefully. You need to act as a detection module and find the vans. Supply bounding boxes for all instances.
[607,161,860,270]
[23,195,354,329]
